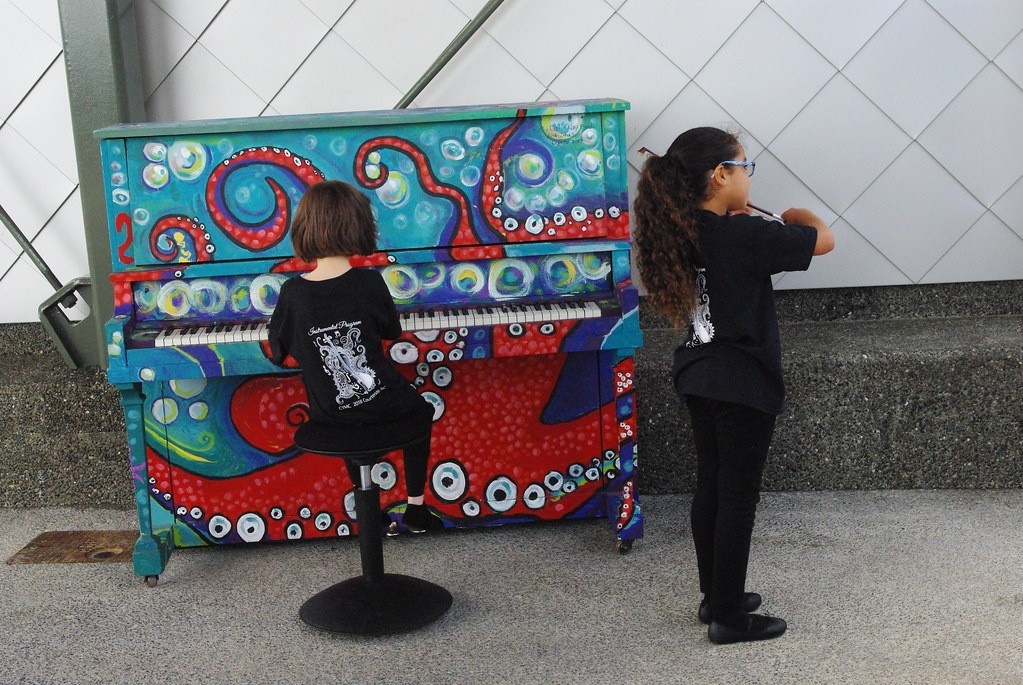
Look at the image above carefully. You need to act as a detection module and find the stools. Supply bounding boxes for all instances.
[294,419,453,635]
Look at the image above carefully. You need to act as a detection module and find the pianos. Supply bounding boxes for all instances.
[90,93,648,588]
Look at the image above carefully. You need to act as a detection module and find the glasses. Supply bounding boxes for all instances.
[710,160,756,181]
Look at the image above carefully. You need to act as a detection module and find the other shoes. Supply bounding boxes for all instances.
[400,504,445,533]
[382,511,391,527]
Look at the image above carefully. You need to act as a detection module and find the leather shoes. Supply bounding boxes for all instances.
[699,592,787,644]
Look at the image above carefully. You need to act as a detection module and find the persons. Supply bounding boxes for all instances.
[267,180,442,533]
[633,126,836,645]
